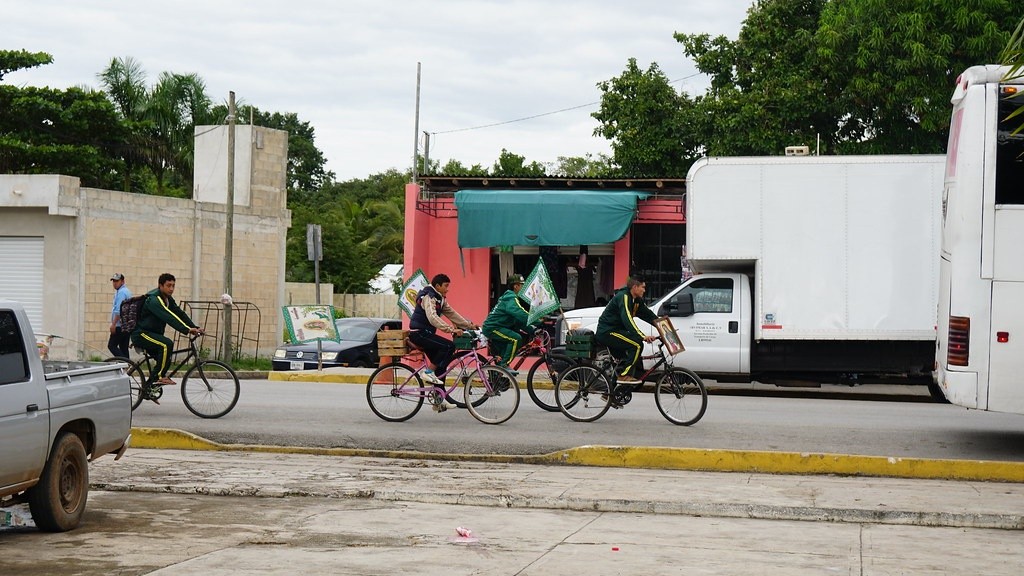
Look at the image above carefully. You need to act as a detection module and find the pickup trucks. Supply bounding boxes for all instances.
[0,302,135,534]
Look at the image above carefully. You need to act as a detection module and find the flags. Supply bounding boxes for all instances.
[518,259,561,326]
[397,266,429,320]
[281,305,342,345]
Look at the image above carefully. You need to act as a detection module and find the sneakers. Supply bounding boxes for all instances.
[600,394,625,410]
[420,370,444,385]
[432,398,457,411]
[616,375,643,384]
[504,366,520,376]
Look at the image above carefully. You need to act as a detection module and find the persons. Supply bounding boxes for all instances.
[597,275,660,410]
[130,273,207,405]
[409,273,478,410]
[107,273,132,360]
[482,273,544,396]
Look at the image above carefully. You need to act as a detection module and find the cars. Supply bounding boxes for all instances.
[271,318,402,371]
[933,64,1024,415]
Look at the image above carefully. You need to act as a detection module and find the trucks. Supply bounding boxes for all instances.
[545,146,950,406]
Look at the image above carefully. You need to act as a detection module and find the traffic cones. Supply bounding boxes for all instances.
[375,323,398,372]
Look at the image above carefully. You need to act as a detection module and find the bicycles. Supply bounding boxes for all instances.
[102,330,241,420]
[365,324,521,425]
[445,315,583,414]
[555,330,709,426]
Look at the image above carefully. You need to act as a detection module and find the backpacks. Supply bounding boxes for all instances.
[120,295,145,335]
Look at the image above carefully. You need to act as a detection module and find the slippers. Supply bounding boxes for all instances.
[153,377,177,386]
[149,396,161,405]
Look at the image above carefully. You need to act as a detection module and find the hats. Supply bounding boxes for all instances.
[110,274,125,281]
[507,274,525,285]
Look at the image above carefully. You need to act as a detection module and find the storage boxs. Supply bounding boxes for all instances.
[566,335,593,357]
[454,330,486,350]
[377,330,410,356]
